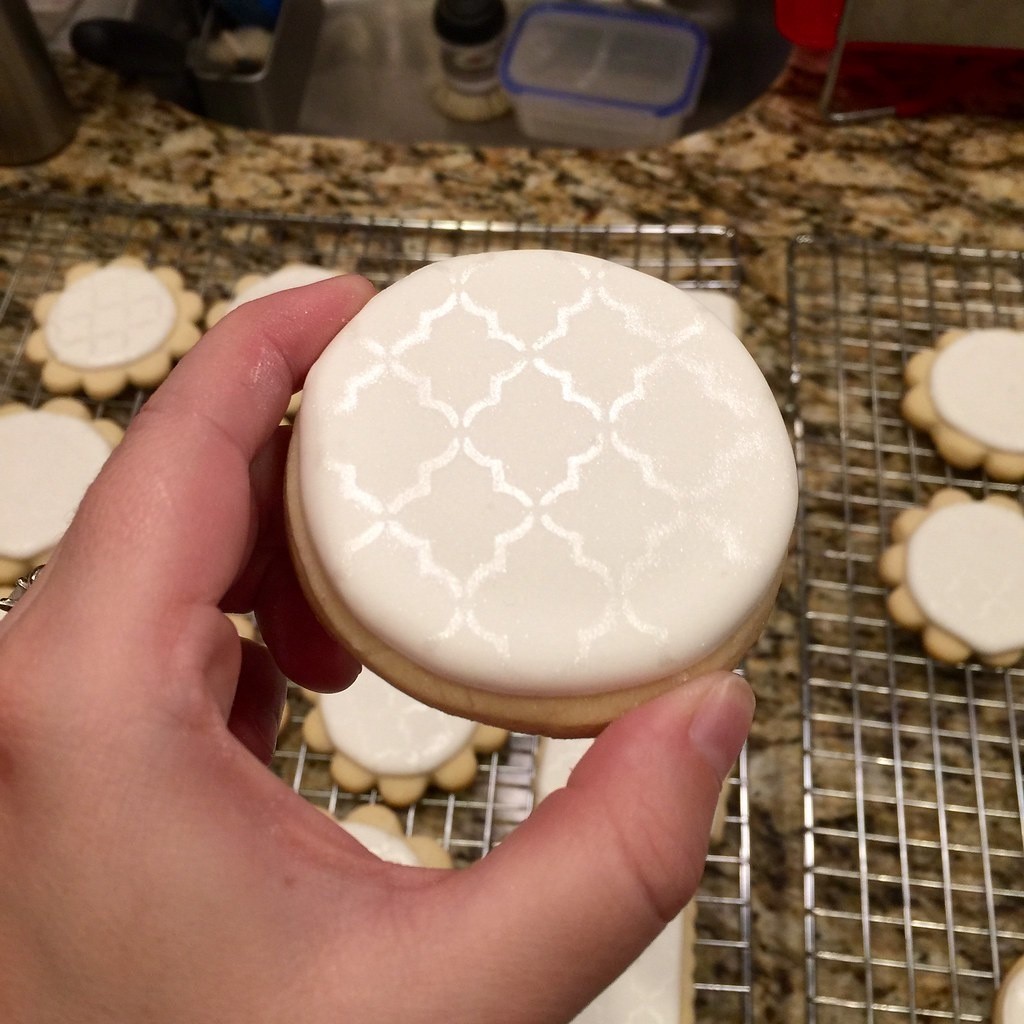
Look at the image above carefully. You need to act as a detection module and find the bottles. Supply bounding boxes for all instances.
[431,0,517,147]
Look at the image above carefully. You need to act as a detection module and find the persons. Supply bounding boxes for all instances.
[0,275,755,1024]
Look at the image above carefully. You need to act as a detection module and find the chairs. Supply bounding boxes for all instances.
[773,0,1024,129]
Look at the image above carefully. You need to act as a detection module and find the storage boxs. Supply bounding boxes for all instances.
[499,1,709,150]
[132,0,322,136]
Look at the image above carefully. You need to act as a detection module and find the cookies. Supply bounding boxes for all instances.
[0,249,1024,1024]
